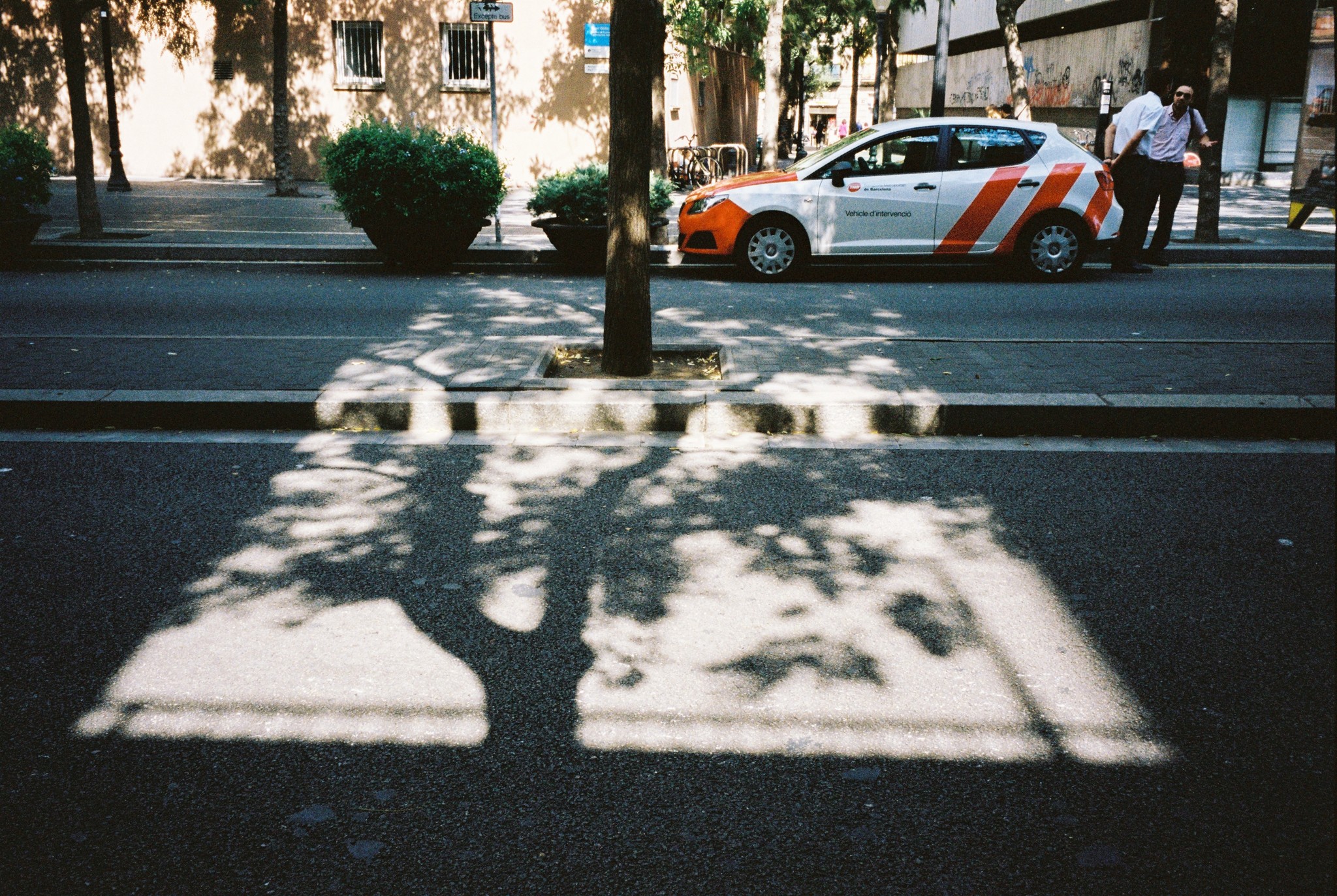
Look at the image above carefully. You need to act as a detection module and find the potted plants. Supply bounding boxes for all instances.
[309,117,505,267]
[529,160,674,268]
[2,122,53,247]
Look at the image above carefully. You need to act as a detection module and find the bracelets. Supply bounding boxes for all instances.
[1104,156,1113,161]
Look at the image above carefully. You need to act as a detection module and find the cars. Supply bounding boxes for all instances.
[678,116,1125,282]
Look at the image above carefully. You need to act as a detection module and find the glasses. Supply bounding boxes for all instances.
[1176,91,1191,100]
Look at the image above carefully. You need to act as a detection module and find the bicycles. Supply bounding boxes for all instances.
[1069,129,1095,152]
[668,134,724,191]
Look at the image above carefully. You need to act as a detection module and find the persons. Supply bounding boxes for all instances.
[814,120,869,151]
[1000,103,1016,118]
[1102,69,1175,275]
[1129,78,1217,275]
[985,103,1004,120]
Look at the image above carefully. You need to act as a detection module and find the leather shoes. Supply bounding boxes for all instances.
[1111,260,1130,273]
[1128,259,1154,272]
[1148,247,1169,266]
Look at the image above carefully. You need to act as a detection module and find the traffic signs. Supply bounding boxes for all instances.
[470,2,513,22]
